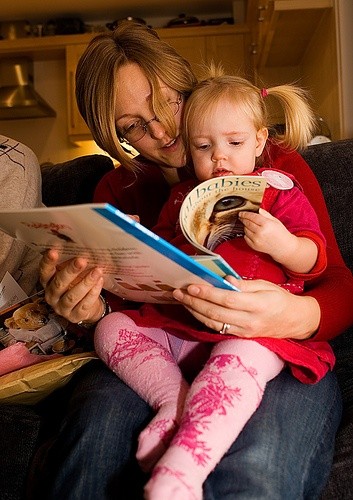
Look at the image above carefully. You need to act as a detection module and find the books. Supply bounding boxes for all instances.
[0,289,95,363]
[0,175,267,304]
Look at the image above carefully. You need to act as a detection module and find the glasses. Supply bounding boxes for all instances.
[117,94,183,144]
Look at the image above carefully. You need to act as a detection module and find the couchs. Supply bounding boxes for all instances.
[0,138,353,500]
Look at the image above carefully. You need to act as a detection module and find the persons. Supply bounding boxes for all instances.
[37,18,353,500]
[94,60,332,500]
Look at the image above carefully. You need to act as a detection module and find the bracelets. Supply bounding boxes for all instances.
[77,294,110,325]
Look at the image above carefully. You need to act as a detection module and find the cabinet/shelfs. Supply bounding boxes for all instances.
[0,0,353,148]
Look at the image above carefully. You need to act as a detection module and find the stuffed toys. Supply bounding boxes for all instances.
[3,299,84,356]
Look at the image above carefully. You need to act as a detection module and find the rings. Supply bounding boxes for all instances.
[220,323,230,335]
